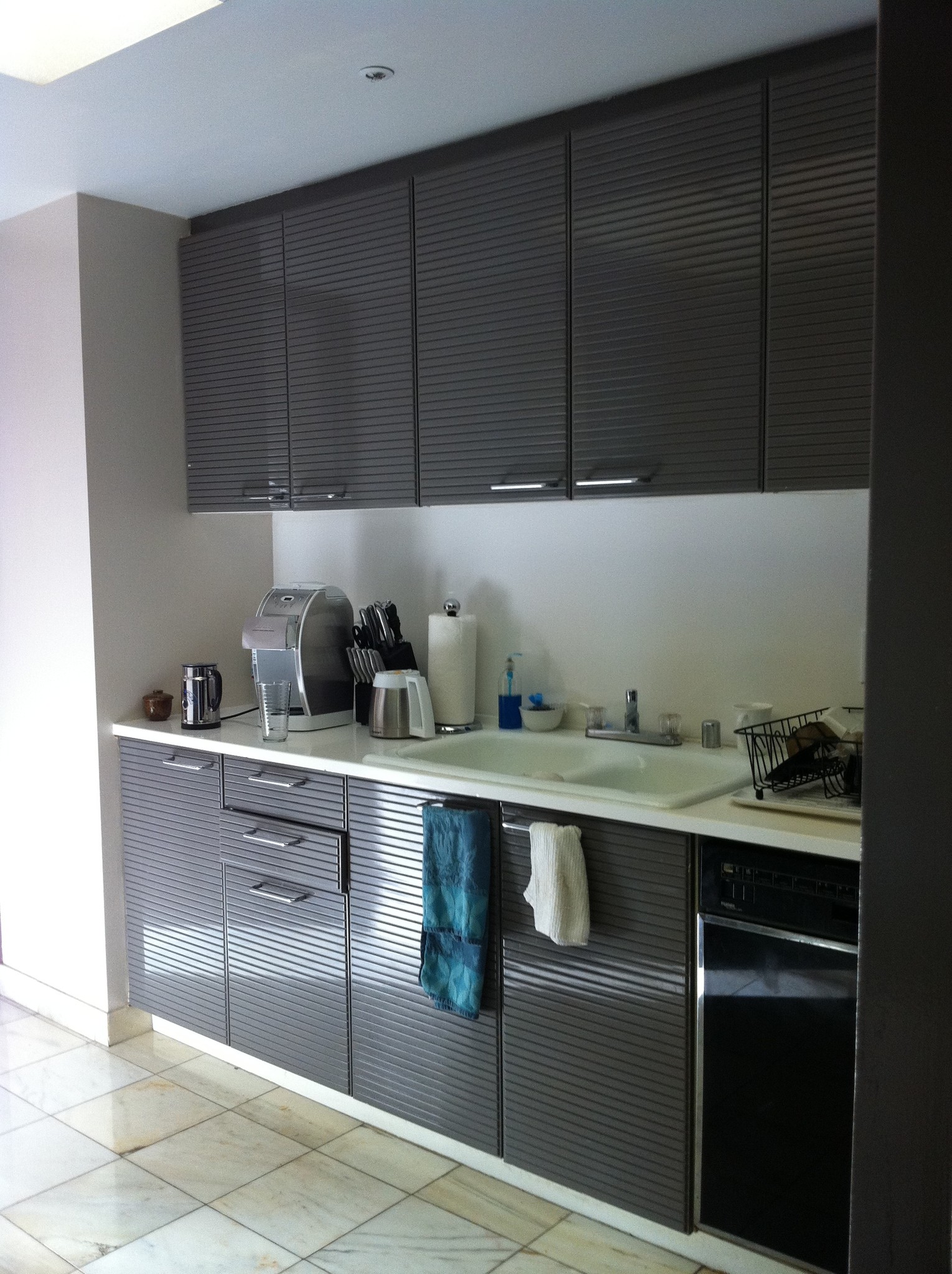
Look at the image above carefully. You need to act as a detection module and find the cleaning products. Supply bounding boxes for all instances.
[497,651,523,729]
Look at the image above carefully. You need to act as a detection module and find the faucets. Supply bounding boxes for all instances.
[583,690,684,746]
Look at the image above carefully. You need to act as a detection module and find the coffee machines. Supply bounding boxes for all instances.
[242,581,354,730]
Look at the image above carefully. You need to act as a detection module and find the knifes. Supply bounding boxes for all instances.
[346,600,404,685]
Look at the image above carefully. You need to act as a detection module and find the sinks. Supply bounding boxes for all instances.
[558,741,774,809]
[364,725,628,787]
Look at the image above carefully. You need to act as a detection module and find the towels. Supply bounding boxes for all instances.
[523,820,590,947]
[415,802,494,1021]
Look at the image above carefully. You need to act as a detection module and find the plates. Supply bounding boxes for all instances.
[764,742,820,782]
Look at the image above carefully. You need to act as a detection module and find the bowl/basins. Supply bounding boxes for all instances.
[787,721,840,758]
[519,705,566,732]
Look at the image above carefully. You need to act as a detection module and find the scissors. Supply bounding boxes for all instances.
[352,625,373,649]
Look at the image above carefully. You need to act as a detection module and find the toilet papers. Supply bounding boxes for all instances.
[426,612,477,726]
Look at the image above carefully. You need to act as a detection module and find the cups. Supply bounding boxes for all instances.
[733,701,774,755]
[181,662,223,730]
[818,706,863,739]
[256,679,292,743]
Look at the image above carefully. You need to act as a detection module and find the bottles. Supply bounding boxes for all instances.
[701,720,720,748]
[143,689,174,721]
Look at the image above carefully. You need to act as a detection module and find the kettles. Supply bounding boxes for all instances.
[369,669,436,739]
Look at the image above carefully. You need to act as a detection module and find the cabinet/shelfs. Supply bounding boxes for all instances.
[343,778,691,1242]
[415,74,769,512]
[114,735,228,1047]
[220,753,353,1100]
[178,181,417,514]
[759,49,879,497]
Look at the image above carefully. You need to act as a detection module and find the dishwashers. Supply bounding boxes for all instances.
[693,834,860,1274]
